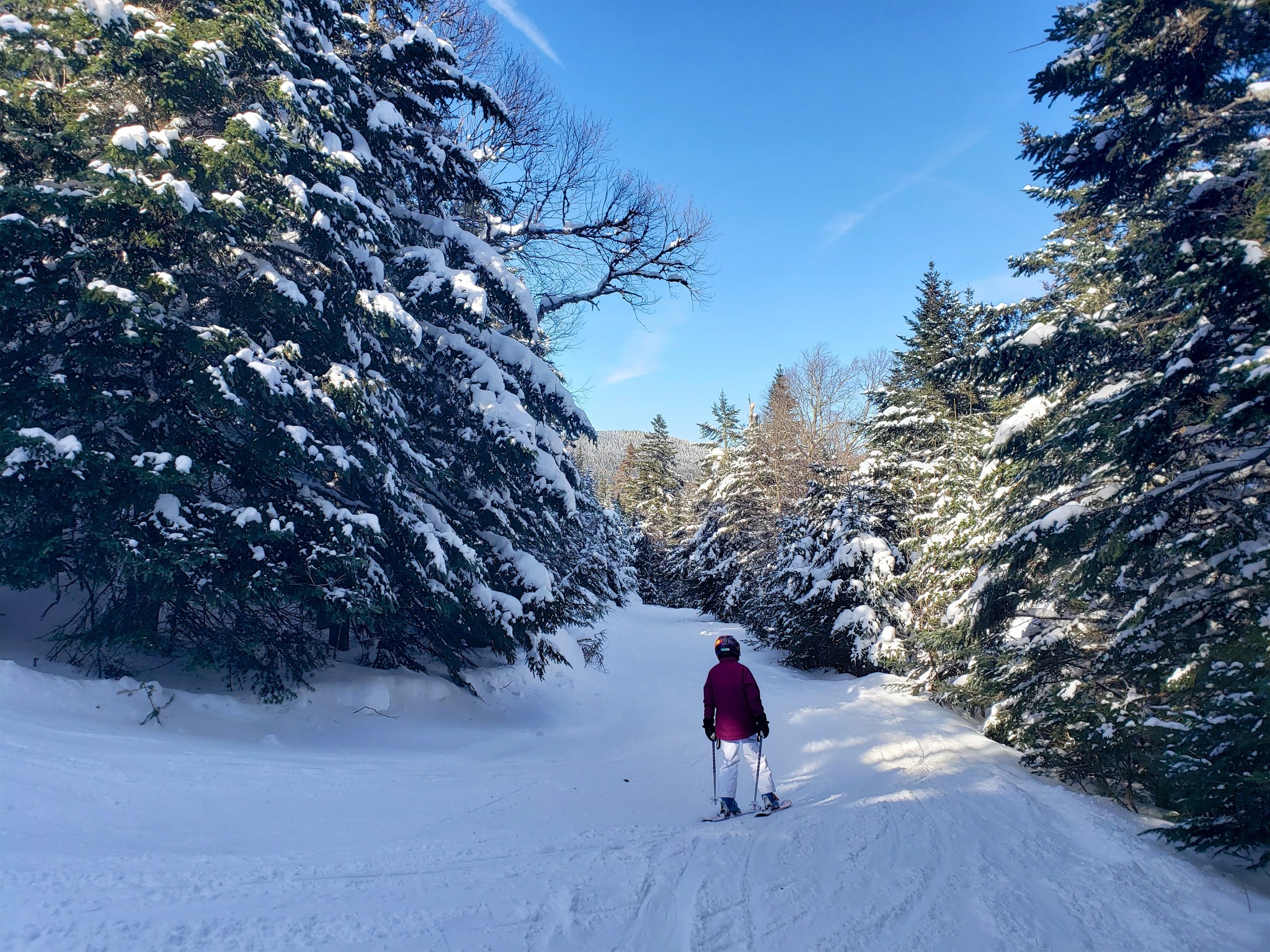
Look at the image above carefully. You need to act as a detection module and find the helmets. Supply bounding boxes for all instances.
[714,635,741,658]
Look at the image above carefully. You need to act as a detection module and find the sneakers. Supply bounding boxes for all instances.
[762,793,779,809]
[720,797,741,814]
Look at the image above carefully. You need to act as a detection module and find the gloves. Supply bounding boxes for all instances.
[756,713,770,739]
[702,717,718,742]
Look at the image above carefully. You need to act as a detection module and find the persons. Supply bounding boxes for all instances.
[702,635,792,821]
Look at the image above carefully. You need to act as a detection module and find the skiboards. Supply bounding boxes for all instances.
[702,800,792,822]
